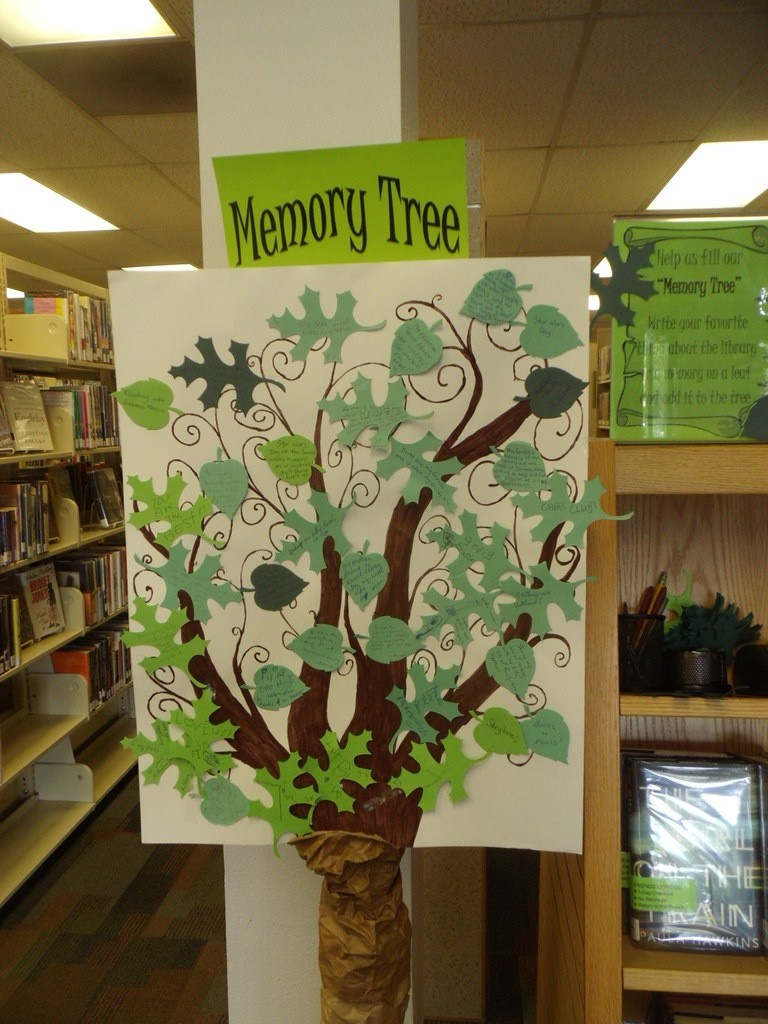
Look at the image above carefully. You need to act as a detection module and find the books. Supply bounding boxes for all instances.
[0,289,132,716]
[618,745,768,956]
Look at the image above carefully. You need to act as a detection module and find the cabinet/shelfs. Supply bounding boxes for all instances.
[530,325,768,1024]
[0,253,130,923]
